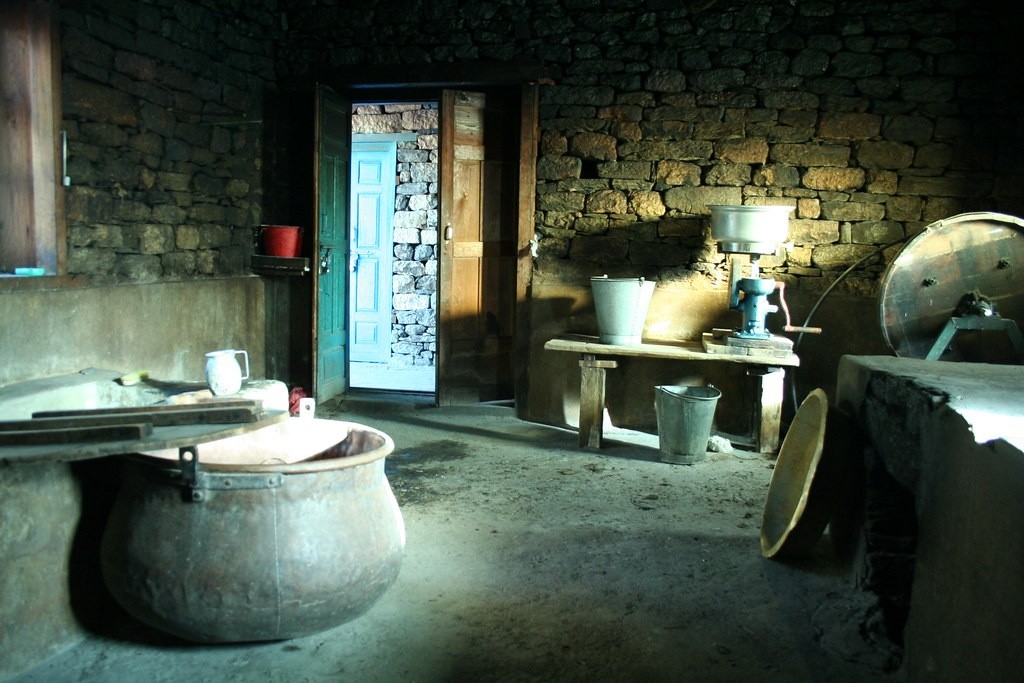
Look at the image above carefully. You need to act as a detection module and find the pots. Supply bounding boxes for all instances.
[68,419,406,642]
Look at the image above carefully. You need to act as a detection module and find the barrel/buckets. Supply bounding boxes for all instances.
[588,278,656,345]
[654,384,722,463]
[253,224,300,257]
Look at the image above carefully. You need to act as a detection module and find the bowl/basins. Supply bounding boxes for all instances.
[707,204,796,242]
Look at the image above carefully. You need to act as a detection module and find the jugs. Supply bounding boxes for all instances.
[204,349,250,397]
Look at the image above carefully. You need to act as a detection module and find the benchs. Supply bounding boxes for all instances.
[542,333,800,453]
